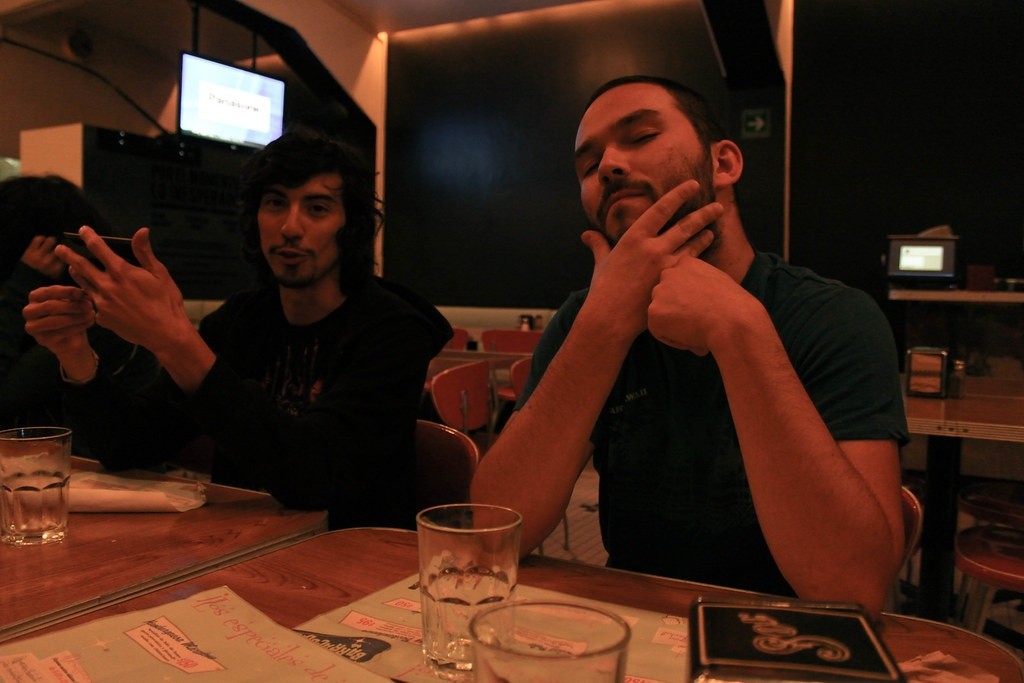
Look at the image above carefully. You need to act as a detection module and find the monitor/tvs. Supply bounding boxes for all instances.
[175,50,288,153]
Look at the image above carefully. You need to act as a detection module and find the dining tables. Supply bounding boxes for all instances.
[2,435,333,636]
[0,525,1023,682]
[901,369,1024,624]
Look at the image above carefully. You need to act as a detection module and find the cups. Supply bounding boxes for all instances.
[0,427,71,546]
[468,598,633,683]
[417,504,521,678]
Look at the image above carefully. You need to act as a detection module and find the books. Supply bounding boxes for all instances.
[688,596,908,683]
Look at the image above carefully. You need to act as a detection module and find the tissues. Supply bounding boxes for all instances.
[886,223,968,286]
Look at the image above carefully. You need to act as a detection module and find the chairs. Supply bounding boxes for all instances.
[411,326,571,560]
[897,481,1023,654]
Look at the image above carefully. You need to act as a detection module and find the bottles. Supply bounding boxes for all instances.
[517,314,543,330]
[948,359,967,398]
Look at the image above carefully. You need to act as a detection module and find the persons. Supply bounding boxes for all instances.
[1,172,160,463]
[22,126,456,534]
[467,74,913,625]
